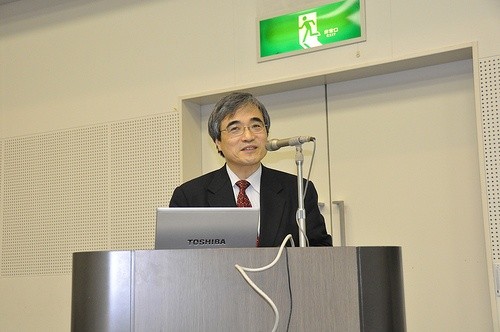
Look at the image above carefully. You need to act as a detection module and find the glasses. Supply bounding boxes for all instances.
[218,122,269,136]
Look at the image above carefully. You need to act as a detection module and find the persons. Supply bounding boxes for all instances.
[168,91,333,247]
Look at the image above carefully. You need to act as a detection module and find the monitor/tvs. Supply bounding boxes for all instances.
[155,206,259,248]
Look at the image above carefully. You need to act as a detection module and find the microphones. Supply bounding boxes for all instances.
[265,136,315,152]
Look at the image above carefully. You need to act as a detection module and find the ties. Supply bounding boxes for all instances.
[234,180,260,246]
